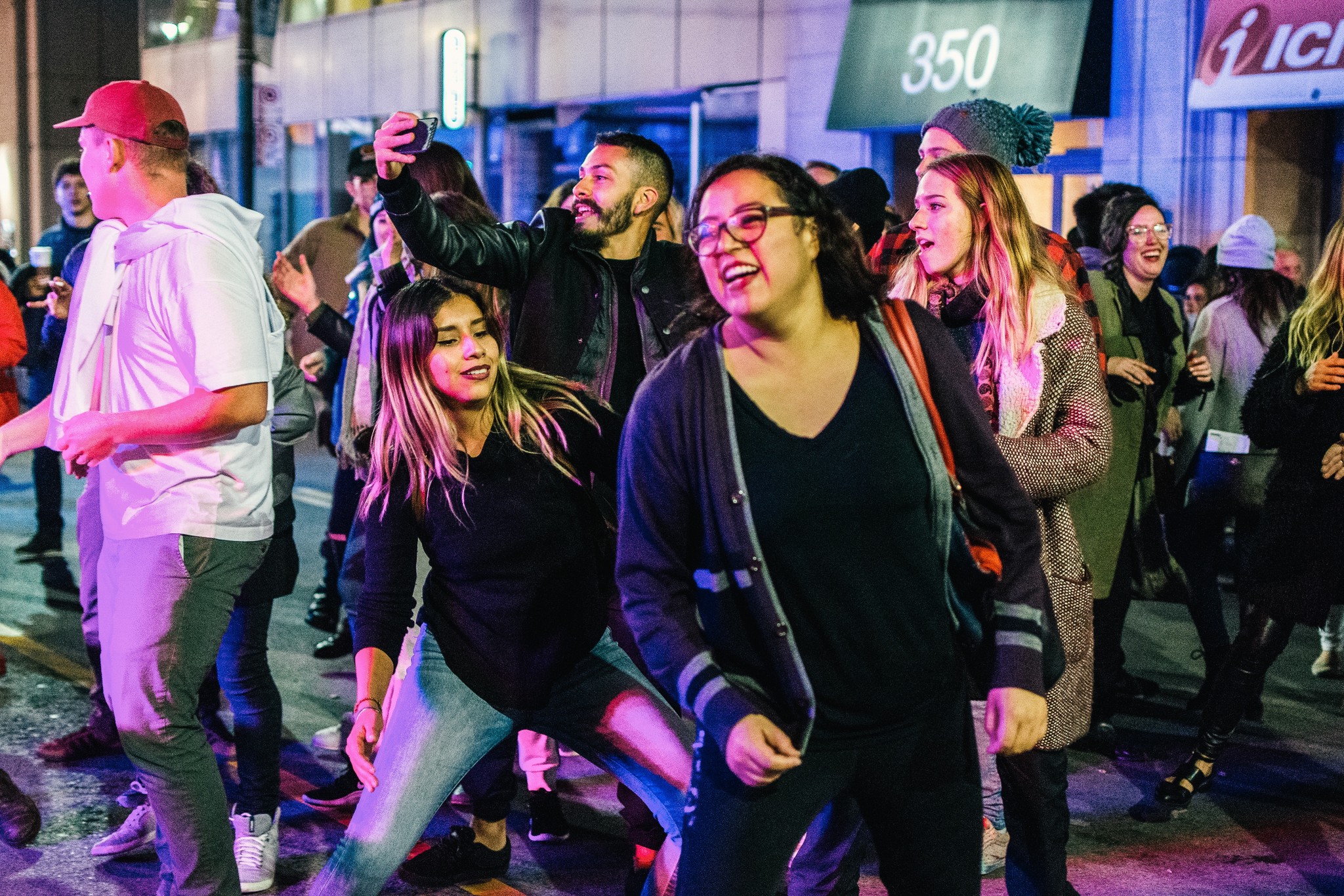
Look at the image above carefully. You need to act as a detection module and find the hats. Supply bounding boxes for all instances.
[52,79,189,149]
[1216,215,1278,270]
[921,99,1055,173]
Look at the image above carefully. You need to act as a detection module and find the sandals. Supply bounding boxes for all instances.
[1243,695,1263,719]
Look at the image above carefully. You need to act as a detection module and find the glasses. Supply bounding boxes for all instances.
[688,206,815,255]
[1126,223,1172,242]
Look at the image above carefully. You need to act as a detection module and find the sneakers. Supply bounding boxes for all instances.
[303,763,364,805]
[397,824,512,886]
[89,777,158,856]
[33,725,111,761]
[229,802,281,893]
[200,714,237,758]
[527,786,571,842]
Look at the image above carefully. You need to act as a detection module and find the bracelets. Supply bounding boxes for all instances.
[353,696,382,721]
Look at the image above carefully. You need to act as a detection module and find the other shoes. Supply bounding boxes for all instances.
[1155,747,1215,806]
[1191,649,1219,708]
[116,781,146,807]
[313,633,352,659]
[980,820,1010,874]
[1311,649,1338,676]
[15,531,62,555]
[511,746,526,779]
[305,582,341,632]
[312,722,342,753]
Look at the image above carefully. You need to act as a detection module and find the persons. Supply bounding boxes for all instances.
[0,282,42,846]
[868,101,1110,875]
[1155,214,1344,807]
[372,110,730,896]
[1169,215,1305,718]
[1177,243,1240,588]
[1056,196,1217,754]
[1311,605,1344,679]
[1066,185,1146,271]
[51,79,285,896]
[14,161,130,760]
[302,279,698,896]
[91,165,318,893]
[885,153,1115,896]
[267,143,573,843]
[617,154,1047,896]
[1272,248,1310,300]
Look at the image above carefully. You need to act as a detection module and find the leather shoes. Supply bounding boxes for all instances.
[0,766,40,847]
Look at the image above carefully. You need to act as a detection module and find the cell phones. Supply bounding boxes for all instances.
[393,117,438,155]
